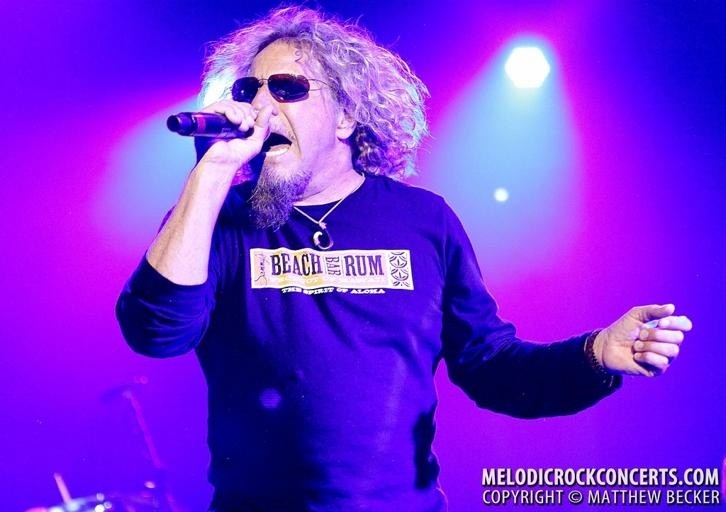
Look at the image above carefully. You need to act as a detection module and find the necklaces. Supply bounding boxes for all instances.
[291,167,366,250]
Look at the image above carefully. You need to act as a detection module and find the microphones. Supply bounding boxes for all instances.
[165,111,254,138]
[99,379,147,404]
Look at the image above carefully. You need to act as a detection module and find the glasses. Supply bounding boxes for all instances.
[231,74,332,104]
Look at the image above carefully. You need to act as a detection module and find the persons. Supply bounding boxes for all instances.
[110,1,695,510]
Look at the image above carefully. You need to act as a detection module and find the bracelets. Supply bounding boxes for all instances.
[586,324,616,390]
